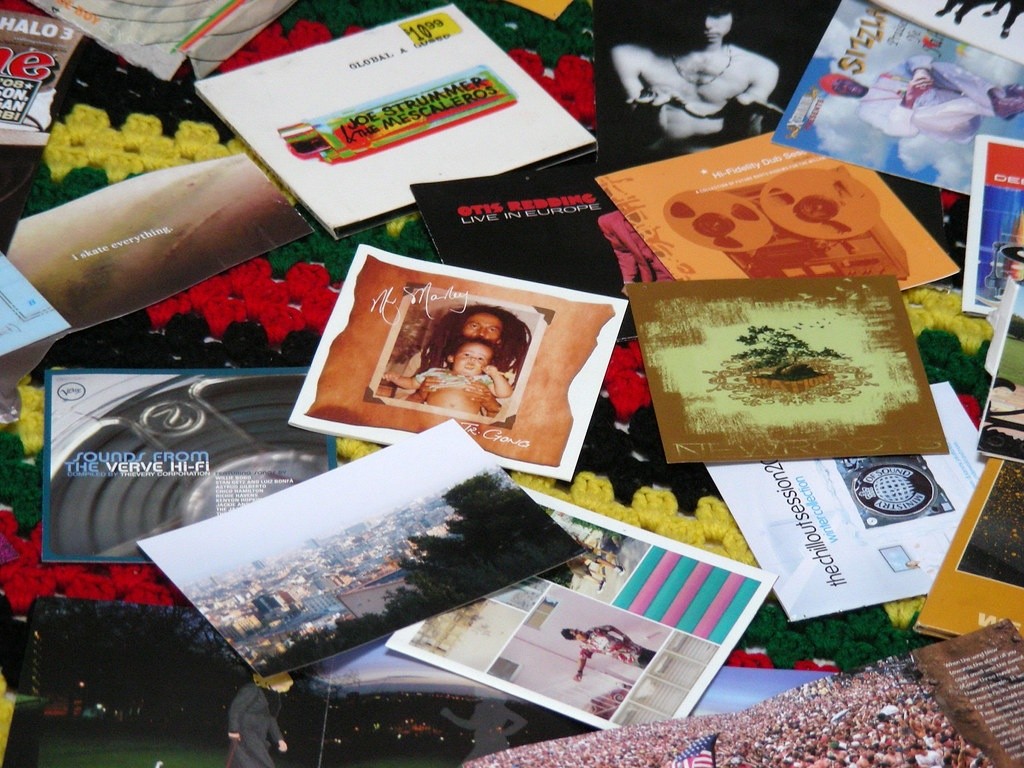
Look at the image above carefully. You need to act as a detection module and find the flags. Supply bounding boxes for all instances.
[670,733,719,768]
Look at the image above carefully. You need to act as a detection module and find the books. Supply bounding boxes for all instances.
[0,9,84,146]
[194,3,598,239]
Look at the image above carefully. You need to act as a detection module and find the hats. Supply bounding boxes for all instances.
[819,73,849,96]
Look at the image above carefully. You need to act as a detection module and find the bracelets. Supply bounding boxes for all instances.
[578,671,582,673]
[561,625,656,682]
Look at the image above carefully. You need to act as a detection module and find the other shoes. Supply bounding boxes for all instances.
[1003,84,1024,97]
[991,96,1024,119]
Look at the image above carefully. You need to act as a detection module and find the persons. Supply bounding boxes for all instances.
[819,56,1024,145]
[383,305,532,418]
[226,683,287,768]
[460,656,994,768]
[611,0,779,164]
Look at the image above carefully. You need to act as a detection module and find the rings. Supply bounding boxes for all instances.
[922,83,927,87]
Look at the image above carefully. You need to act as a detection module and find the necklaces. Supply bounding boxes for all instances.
[671,44,732,85]
[861,87,902,101]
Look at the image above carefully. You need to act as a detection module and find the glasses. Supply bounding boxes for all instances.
[575,629,579,637]
